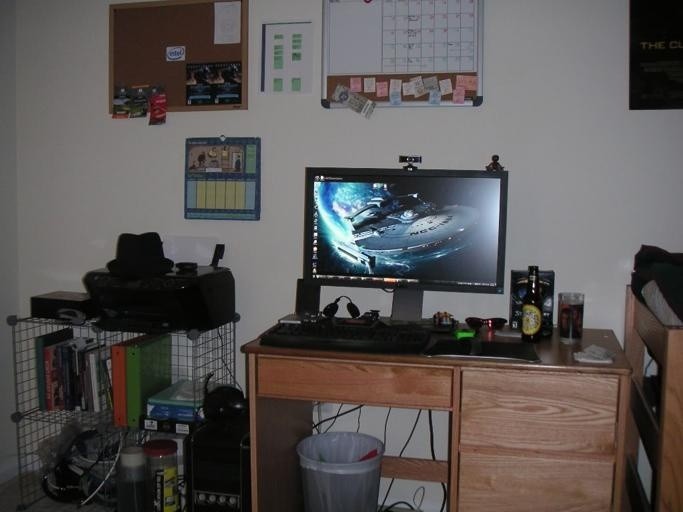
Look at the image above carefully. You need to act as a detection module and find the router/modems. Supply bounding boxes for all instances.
[279,312,322,324]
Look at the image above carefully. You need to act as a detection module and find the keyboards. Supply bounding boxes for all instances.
[259,324,431,354]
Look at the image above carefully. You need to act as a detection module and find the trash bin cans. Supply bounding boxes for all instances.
[296,431,385,512]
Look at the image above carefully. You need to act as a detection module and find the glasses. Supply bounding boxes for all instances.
[466,318,506,330]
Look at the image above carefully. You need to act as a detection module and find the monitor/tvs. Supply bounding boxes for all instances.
[304,167,508,332]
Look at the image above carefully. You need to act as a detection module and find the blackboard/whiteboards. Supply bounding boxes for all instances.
[321,0,484,109]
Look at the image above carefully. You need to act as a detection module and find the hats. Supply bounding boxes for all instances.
[106,234,173,276]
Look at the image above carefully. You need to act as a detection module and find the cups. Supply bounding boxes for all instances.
[554,292,589,346]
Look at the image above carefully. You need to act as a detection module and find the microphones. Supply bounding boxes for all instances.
[204,372,214,396]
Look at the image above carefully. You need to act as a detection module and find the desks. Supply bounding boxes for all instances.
[239,315,634,512]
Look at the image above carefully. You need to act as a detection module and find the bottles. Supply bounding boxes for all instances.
[517,266,542,342]
[116,444,149,511]
[142,439,181,512]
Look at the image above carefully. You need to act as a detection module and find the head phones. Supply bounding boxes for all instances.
[202,386,248,426]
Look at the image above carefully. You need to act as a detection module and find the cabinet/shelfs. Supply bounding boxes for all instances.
[4,311,240,512]
[620,284,681,511]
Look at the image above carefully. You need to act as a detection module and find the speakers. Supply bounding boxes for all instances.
[296,278,320,314]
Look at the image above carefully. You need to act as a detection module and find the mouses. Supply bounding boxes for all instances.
[460,337,481,355]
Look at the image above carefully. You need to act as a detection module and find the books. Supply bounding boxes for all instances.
[34,326,172,430]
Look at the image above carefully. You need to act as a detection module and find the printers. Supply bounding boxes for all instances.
[83,266,235,332]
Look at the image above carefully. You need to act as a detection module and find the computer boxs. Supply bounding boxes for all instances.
[182,422,251,512]
